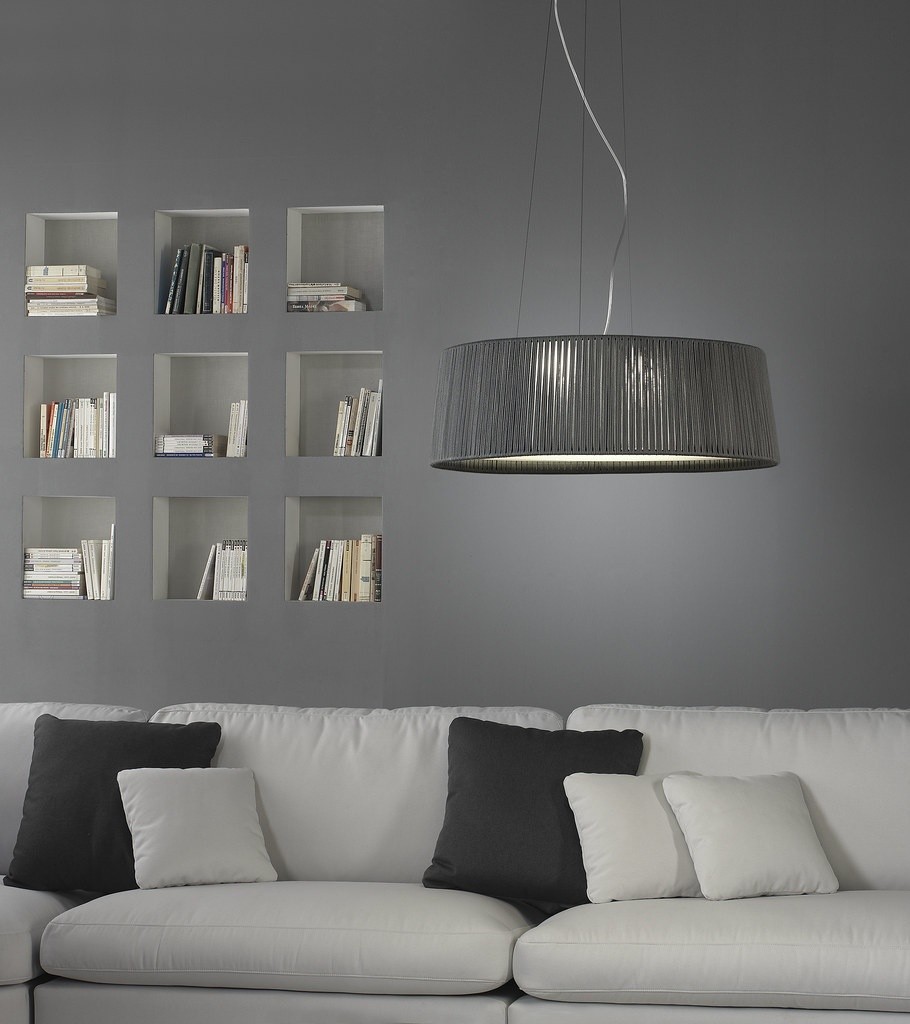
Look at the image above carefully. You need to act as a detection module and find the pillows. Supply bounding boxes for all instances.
[117,766,278,888]
[663,771,840,901]
[2,714,222,892]
[562,772,704,903]
[422,717,643,906]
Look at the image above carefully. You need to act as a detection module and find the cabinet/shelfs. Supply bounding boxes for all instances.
[22,204,383,602]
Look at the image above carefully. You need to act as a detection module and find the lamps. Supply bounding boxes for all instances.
[429,1,779,474]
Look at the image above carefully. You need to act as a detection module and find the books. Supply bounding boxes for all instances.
[334,379,383,456]
[25,264,116,316]
[165,244,248,313]
[23,524,115,600]
[288,282,366,312]
[155,399,248,457]
[299,534,382,602]
[197,540,248,601]
[40,392,116,458]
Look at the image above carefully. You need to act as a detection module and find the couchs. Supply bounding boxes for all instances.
[0,701,909,1024]
